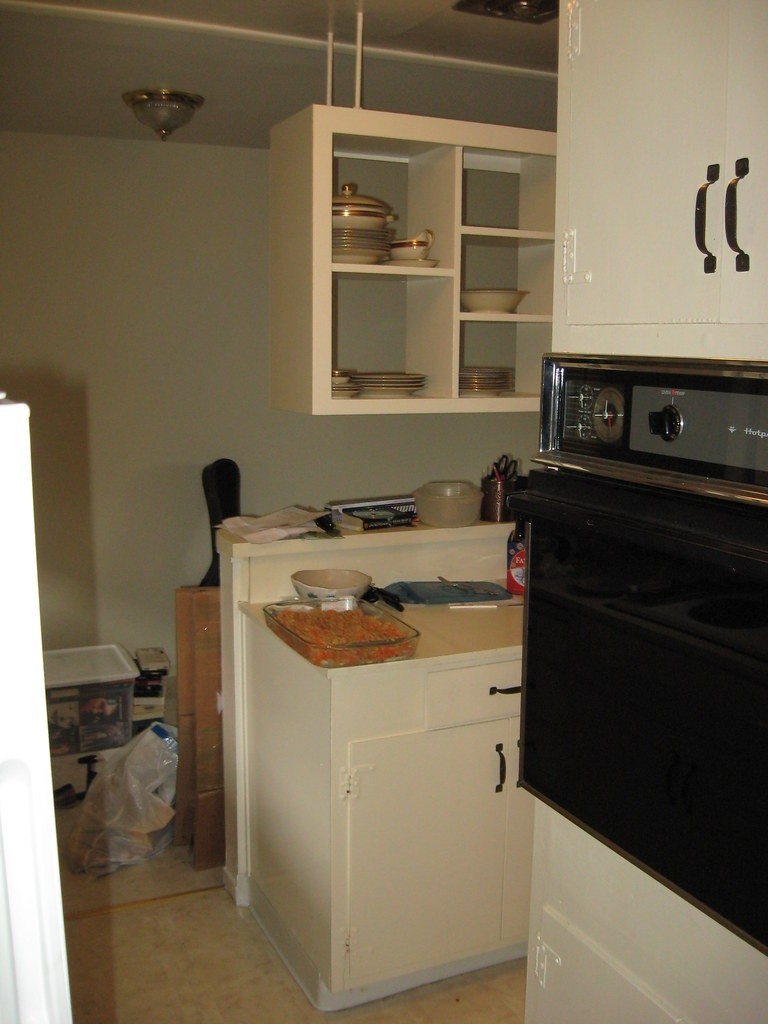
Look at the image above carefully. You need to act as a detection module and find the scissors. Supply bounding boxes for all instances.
[499,455,517,481]
[438,576,474,592]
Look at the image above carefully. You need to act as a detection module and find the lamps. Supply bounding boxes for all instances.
[122,87,206,144]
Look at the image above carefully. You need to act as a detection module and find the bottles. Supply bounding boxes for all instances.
[506,513,526,595]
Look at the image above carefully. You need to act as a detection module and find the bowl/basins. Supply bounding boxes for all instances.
[460,290,530,314]
[332,370,350,384]
[290,570,371,601]
[412,483,484,527]
[389,230,433,260]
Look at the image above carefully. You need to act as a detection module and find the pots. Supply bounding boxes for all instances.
[332,185,394,229]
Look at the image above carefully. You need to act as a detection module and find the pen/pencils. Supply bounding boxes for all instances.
[481,463,501,481]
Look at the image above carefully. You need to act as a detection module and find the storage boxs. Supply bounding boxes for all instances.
[42,641,165,757]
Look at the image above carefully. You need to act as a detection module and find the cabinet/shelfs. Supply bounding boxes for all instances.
[239,574,527,1013]
[269,103,557,417]
[525,797,768,1024]
[549,0,768,364]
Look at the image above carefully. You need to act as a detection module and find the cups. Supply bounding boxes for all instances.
[482,479,515,522]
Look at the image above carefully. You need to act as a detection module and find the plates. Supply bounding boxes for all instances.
[459,367,512,397]
[332,383,360,398]
[381,259,439,267]
[263,597,420,666]
[332,228,395,264]
[350,374,427,398]
